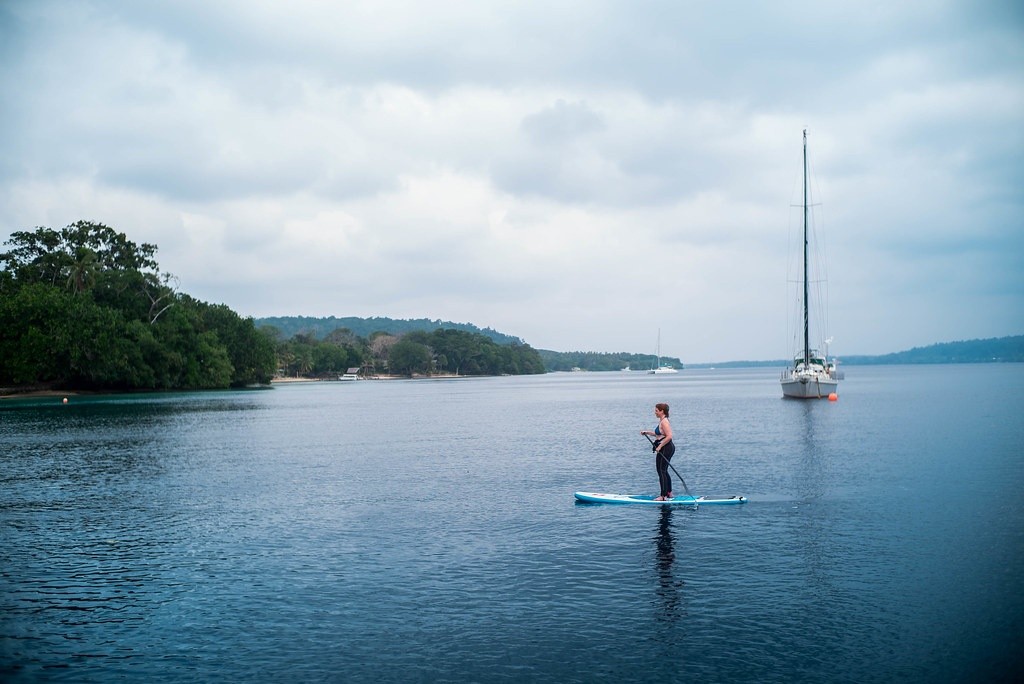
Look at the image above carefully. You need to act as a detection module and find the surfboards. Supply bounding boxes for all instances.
[574,490,748,507]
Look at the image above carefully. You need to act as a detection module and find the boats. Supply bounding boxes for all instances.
[621,366,632,372]
[339,374,359,381]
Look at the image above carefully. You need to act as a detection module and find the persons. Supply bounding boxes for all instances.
[641,403,675,500]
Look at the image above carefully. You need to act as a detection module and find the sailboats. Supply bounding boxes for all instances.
[778,127,844,399]
[645,327,678,374]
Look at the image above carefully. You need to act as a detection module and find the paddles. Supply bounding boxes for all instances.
[642,432,694,498]
